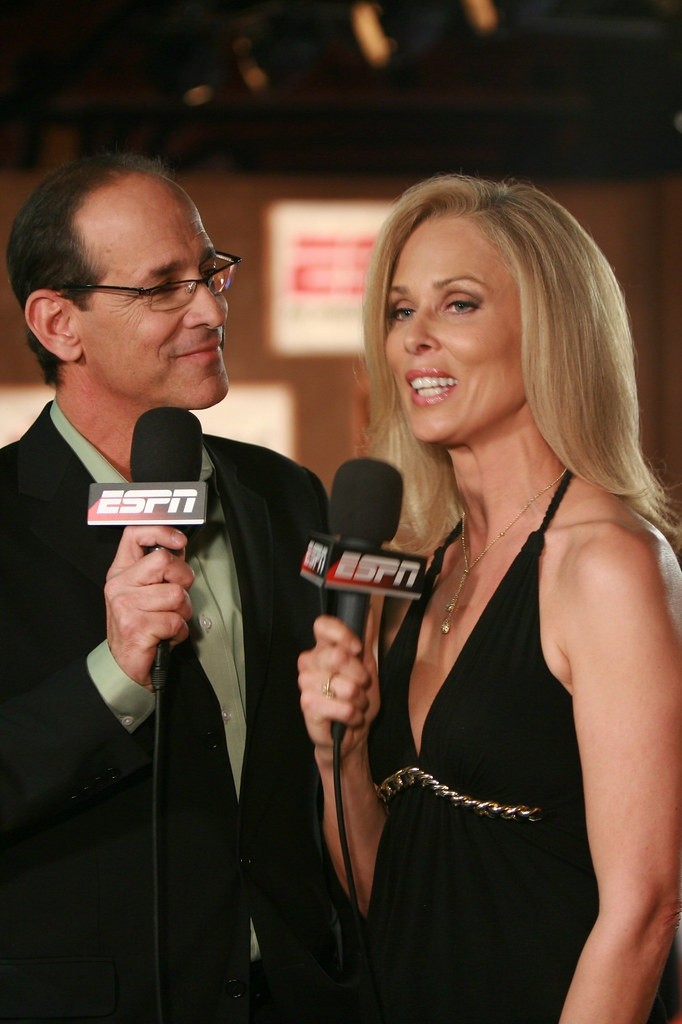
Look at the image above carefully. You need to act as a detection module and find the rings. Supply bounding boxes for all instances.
[321,671,341,700]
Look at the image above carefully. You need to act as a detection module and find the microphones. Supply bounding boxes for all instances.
[301,456,429,742]
[87,405,211,693]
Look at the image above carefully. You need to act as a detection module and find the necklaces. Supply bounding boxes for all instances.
[441,468,567,634]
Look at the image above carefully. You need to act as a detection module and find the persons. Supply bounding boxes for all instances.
[0,153,345,1024]
[298,175,682,1024]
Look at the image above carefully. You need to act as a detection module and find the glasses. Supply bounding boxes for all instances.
[52,250,242,312]
